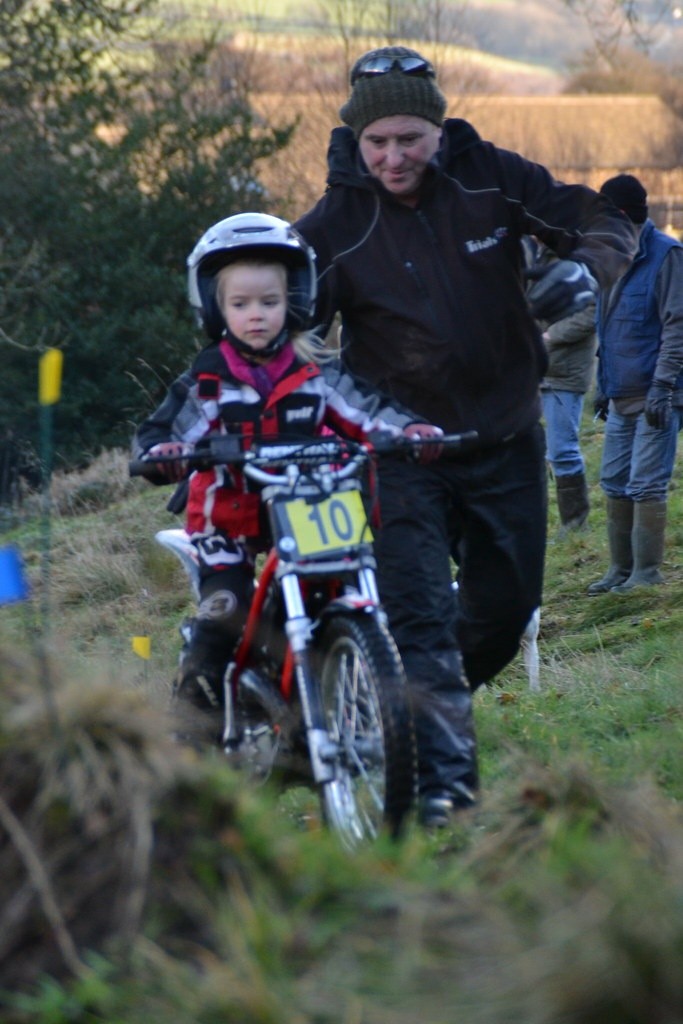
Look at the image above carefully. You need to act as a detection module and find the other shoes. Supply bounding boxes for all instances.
[419,793,469,828]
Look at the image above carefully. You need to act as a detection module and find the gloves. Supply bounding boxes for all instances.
[645,381,675,430]
[594,389,610,418]
[142,440,191,485]
[403,422,445,457]
[525,256,600,324]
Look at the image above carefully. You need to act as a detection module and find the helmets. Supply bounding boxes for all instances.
[186,213,317,331]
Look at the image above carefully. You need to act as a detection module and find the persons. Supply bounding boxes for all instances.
[131,213,444,758]
[293,47,637,831]
[586,174,683,596]
[528,232,598,543]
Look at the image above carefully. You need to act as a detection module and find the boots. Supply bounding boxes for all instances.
[585,494,667,596]
[554,477,591,545]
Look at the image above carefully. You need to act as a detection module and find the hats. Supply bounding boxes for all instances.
[340,47,448,138]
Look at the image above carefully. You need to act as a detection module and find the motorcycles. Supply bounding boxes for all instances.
[125,427,483,857]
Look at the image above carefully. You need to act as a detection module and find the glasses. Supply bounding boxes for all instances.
[352,55,438,80]
